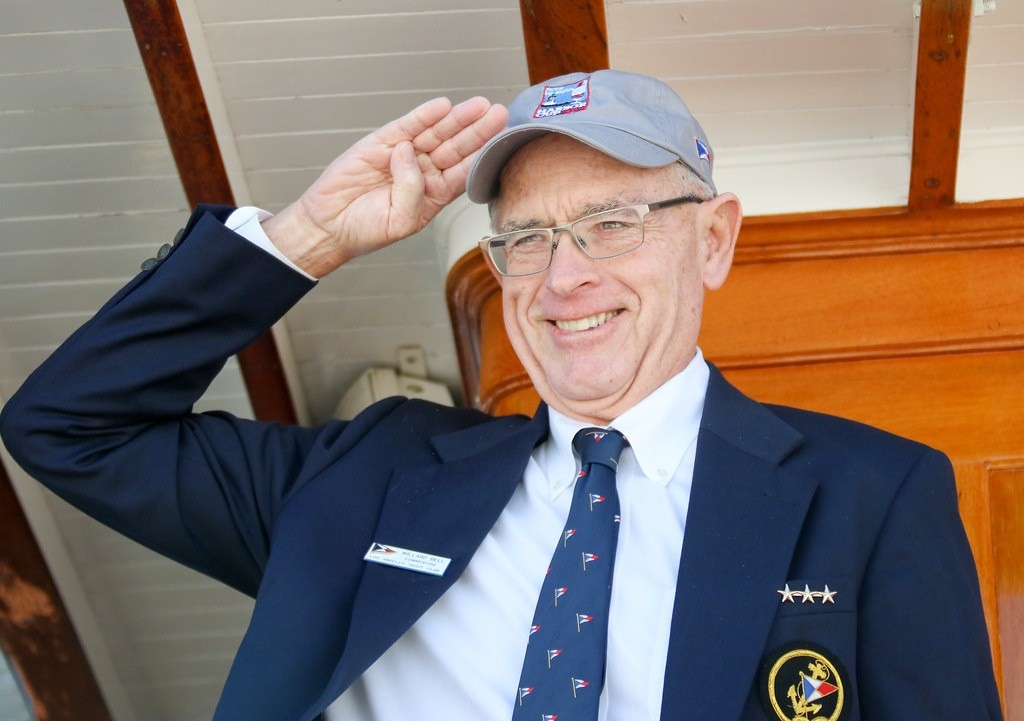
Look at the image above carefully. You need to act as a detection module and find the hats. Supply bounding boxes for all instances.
[466,69,717,205]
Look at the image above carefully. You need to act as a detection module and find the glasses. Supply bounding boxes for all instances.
[478,196,703,277]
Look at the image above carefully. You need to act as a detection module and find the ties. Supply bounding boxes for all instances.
[513,427,629,721]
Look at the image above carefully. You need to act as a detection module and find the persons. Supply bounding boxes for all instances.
[0,69,1006,721]
